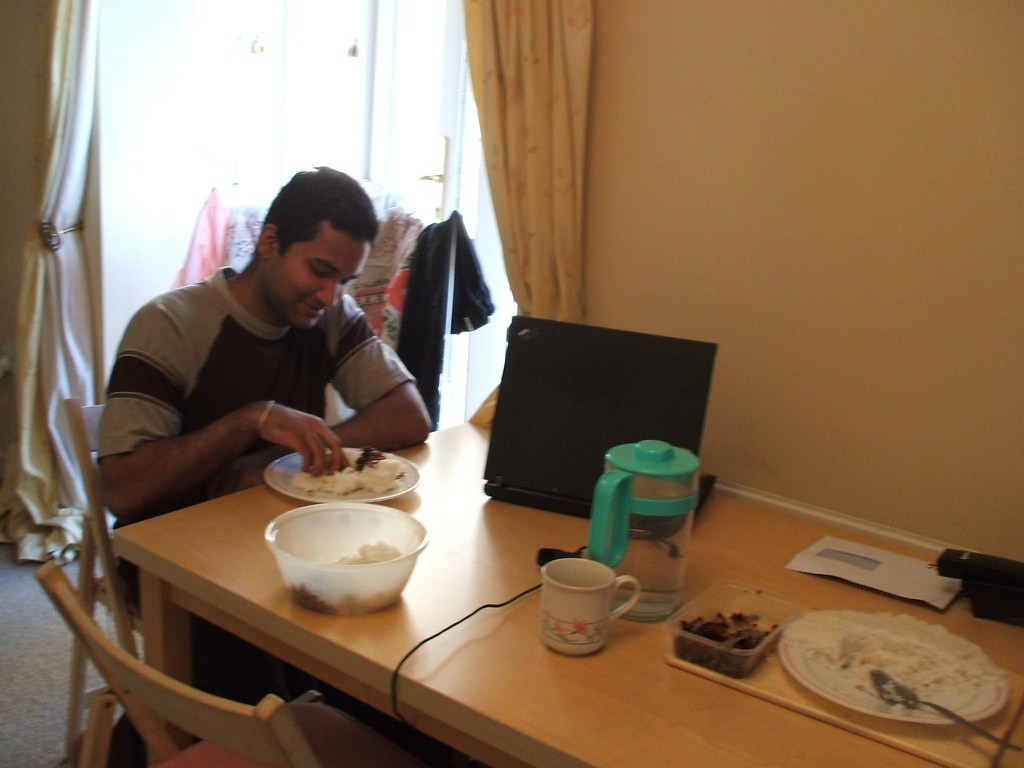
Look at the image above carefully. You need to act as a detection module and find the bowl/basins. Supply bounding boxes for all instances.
[263,502,429,616]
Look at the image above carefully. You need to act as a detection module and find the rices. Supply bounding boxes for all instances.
[292,453,404,495]
[798,618,982,711]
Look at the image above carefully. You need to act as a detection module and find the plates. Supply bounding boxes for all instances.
[262,447,421,502]
[778,613,1009,727]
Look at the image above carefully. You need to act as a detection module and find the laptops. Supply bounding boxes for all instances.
[482,312,720,536]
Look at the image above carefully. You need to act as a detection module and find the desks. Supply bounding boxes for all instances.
[109,420,1023,768]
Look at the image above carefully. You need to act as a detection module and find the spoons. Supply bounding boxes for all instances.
[870,668,1024,755]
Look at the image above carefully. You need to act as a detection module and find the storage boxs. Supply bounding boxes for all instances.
[663,579,804,679]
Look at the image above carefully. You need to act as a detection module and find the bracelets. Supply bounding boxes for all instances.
[256,400,276,431]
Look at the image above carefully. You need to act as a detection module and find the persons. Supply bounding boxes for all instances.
[70,167,430,768]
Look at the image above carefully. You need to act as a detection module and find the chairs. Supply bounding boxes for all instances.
[35,396,433,768]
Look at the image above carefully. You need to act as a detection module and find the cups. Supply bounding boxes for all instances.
[588,439,701,621]
[538,558,642,656]
